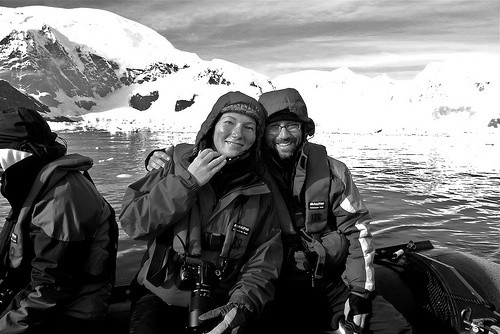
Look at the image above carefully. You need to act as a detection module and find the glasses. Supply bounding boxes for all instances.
[266,121,302,133]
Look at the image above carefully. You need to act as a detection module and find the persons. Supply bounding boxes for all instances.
[0,78,120,334]
[116,88,285,334]
[143,88,374,334]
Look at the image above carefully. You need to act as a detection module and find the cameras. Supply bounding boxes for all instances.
[173,262,232,331]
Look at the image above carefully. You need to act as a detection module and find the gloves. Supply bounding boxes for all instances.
[197,302,259,334]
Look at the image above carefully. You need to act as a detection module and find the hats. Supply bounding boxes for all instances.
[267,109,304,124]
[221,103,261,125]
[0,107,52,154]
